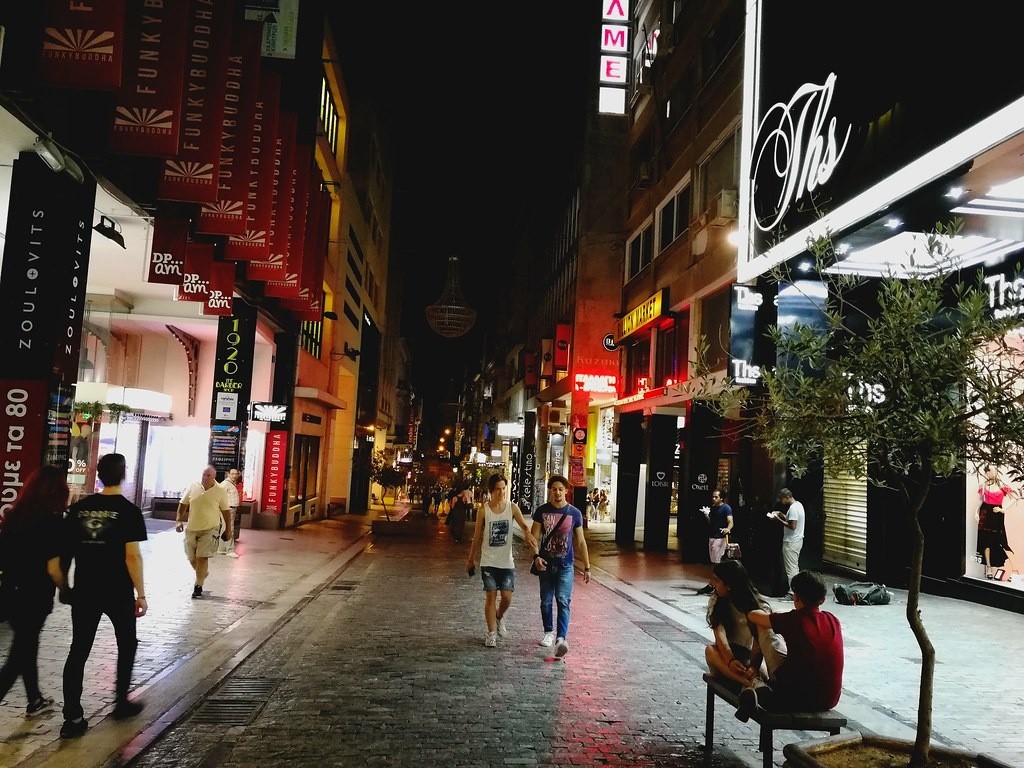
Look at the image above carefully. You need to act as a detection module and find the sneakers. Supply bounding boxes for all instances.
[26,696,55,716]
[554,640,569,657]
[540,631,554,646]
[696,584,717,596]
[192,585,203,598]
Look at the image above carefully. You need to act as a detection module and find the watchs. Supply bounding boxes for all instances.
[533,554,540,561]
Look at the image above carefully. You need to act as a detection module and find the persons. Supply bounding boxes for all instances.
[747,570,844,712]
[175,465,232,599]
[703,559,773,723]
[975,468,1018,582]
[409,485,473,541]
[0,462,69,717]
[531,475,591,658]
[465,474,537,648]
[772,487,805,601]
[697,489,734,595]
[586,487,610,523]
[220,469,240,559]
[57,455,149,739]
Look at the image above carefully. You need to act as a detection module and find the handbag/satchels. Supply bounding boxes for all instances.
[466,502,473,509]
[530,550,544,575]
[833,581,891,605]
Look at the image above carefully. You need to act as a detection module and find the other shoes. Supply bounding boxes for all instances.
[111,701,145,720]
[226,552,238,558]
[60,717,89,739]
[216,550,226,554]
[749,681,773,700]
[496,615,506,637]
[734,689,758,724]
[485,631,499,647]
[779,593,795,602]
[987,574,993,578]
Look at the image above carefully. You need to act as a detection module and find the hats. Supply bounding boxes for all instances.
[777,488,792,499]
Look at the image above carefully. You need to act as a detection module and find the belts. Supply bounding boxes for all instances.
[230,505,236,508]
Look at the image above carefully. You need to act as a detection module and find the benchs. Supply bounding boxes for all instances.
[701,672,850,768]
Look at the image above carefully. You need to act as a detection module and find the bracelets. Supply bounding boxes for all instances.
[585,567,591,571]
[137,596,146,599]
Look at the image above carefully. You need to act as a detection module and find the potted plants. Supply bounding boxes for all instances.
[366,450,409,534]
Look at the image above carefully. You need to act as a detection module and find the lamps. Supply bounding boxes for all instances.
[34,135,65,172]
[63,153,85,184]
[323,311,338,320]
[92,215,127,250]
[330,342,363,363]
[79,301,95,370]
